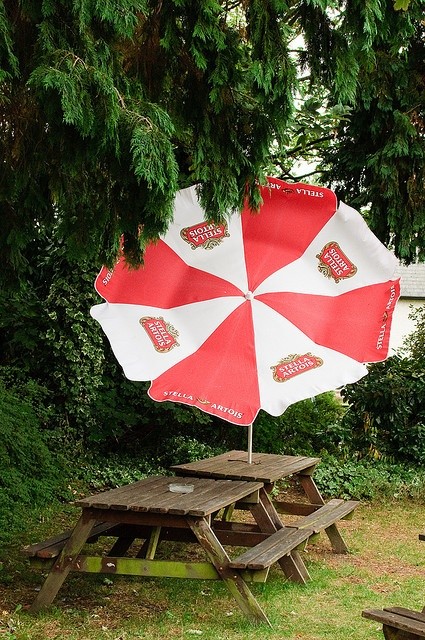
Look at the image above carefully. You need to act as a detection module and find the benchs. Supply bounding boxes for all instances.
[229,526,314,632]
[362,605,424,639]
[288,499,362,582]
[20,520,118,615]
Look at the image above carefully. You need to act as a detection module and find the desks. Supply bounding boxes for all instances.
[27,477,308,629]
[172,448,348,582]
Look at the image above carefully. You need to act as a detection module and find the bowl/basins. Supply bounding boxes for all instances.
[169,483,195,492]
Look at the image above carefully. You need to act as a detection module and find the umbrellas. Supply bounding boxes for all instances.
[90,175,402,464]
